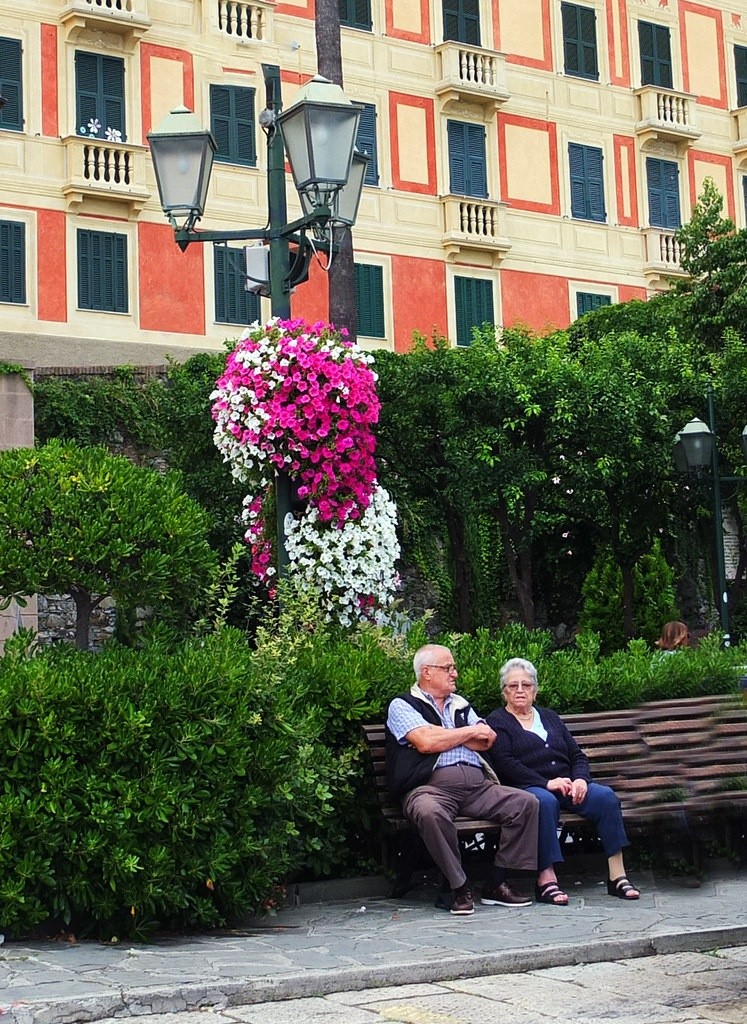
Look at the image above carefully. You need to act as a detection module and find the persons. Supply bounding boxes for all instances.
[660,620,689,654]
[384,644,541,914]
[485,658,640,906]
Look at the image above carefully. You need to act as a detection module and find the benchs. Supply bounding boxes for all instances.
[364,696,746,906]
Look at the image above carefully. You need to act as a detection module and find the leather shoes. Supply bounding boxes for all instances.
[481,880,532,907]
[450,879,475,914]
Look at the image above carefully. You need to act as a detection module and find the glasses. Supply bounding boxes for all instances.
[427,663,457,674]
[504,680,535,690]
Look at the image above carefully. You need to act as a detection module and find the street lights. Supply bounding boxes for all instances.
[147,61,371,576]
[675,385,747,642]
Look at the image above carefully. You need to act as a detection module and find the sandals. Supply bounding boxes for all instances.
[607,876,640,899]
[535,880,568,905]
